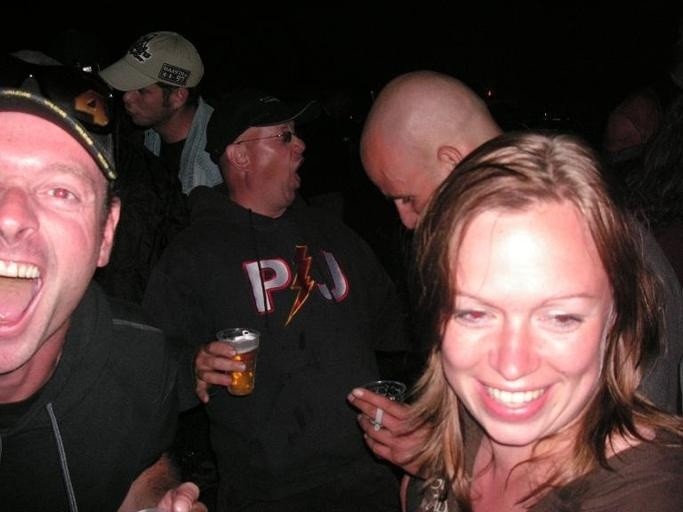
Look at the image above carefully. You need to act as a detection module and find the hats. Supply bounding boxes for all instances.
[0,74,118,184]
[98,31,207,93]
[205,95,318,166]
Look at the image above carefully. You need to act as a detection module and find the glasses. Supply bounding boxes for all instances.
[232,130,295,144]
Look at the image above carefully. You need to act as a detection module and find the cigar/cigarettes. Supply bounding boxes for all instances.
[374,406,384,433]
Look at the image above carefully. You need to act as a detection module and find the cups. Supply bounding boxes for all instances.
[217,327,261,396]
[364,381,407,408]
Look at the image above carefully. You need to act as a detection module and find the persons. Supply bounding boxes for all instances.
[608,22,683,287]
[1,44,213,512]
[98,32,229,231]
[141,91,410,512]
[361,68,683,417]
[389,130,683,511]
